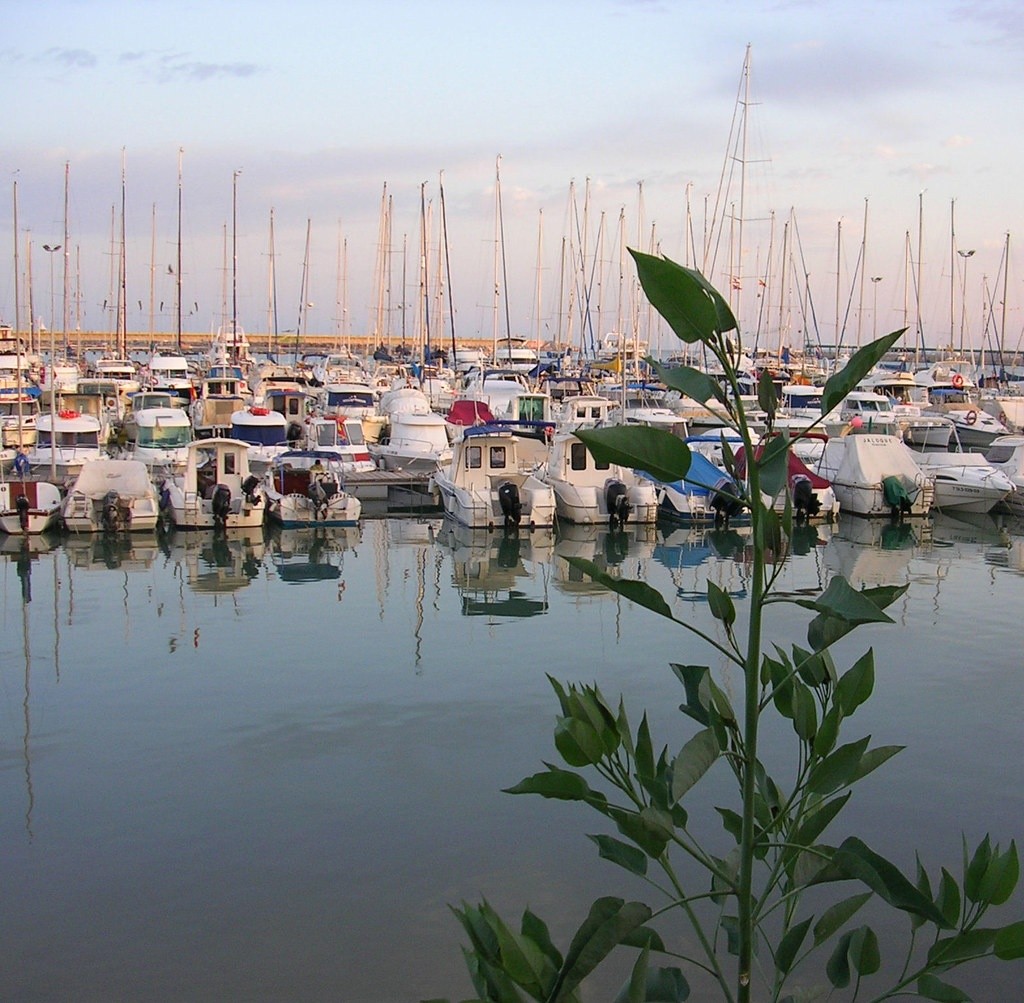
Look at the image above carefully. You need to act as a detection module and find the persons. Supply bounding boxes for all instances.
[851,413,862,434]
[309,460,326,470]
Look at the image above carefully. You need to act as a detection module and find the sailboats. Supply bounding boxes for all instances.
[1,37,1024,536]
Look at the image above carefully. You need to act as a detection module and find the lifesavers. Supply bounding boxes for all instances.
[999,410,1006,424]
[952,375,965,387]
[966,409,977,426]
[252,406,268,415]
[59,409,82,419]
